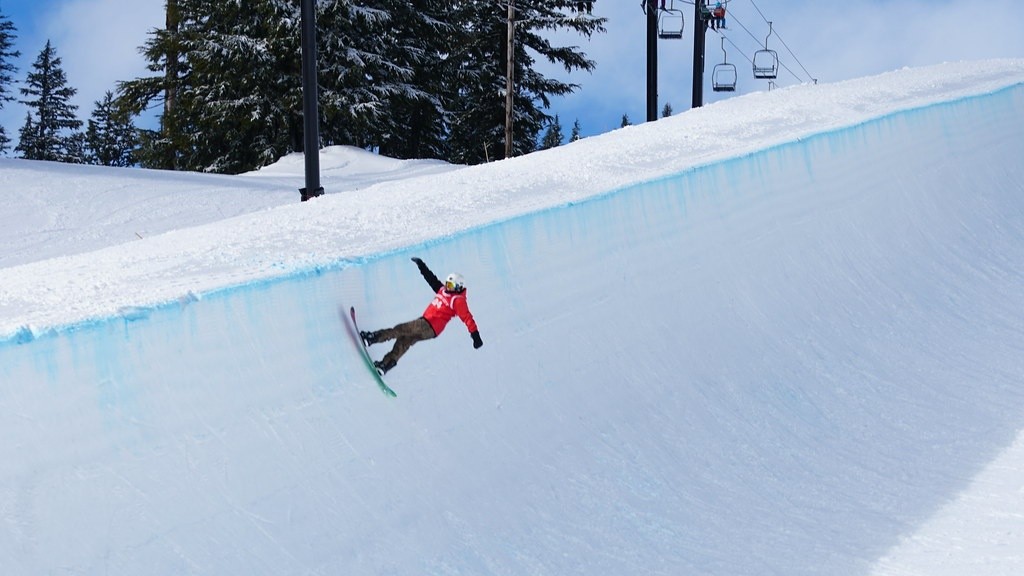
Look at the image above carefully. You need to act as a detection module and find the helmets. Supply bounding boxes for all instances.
[446,273,465,291]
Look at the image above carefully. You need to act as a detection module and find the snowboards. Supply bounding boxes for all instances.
[349,306,398,399]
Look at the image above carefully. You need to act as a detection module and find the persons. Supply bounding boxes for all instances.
[703,1,725,31]
[360,257,483,376]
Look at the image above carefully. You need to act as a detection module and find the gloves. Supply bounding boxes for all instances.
[470,331,483,348]
[411,257,423,266]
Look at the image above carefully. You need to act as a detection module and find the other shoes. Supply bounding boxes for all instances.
[360,330,375,348]
[374,361,387,376]
